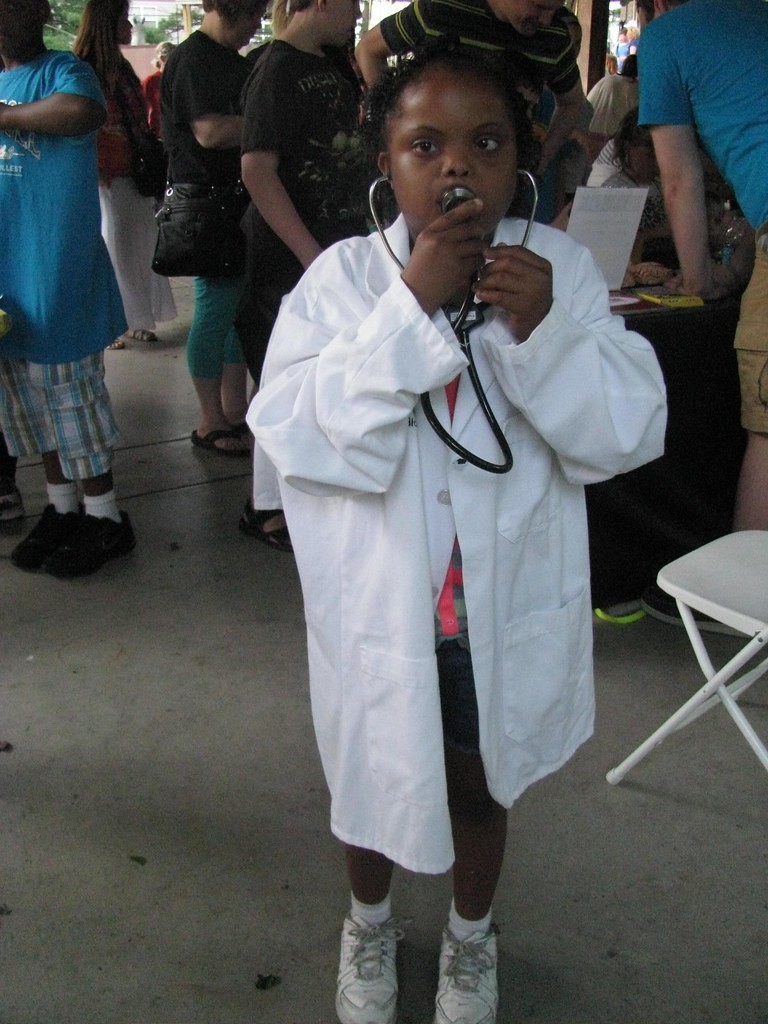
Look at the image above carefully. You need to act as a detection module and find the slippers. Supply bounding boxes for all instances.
[191,420,254,457]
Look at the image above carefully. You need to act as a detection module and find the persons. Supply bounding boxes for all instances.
[72,0,179,349]
[246,31,668,1024]
[636,0,768,638]
[0,0,135,577]
[0,431,27,520]
[141,0,744,551]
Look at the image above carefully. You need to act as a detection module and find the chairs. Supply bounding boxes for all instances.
[606,530,768,785]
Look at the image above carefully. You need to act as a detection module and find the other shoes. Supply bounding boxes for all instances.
[639,588,755,642]
[126,329,157,341]
[106,338,123,350]
[0,488,26,521]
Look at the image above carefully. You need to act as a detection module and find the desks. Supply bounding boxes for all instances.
[582,285,742,610]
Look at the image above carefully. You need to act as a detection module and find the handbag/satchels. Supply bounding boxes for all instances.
[132,136,173,200]
[150,182,250,279]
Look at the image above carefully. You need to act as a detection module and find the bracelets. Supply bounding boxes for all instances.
[726,262,743,286]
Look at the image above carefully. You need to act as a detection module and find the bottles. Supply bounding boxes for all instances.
[723,219,749,266]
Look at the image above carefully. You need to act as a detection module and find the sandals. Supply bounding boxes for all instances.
[239,497,294,553]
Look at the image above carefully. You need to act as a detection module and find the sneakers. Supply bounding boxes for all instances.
[436,922,501,1024]
[10,500,136,576]
[336,910,403,1024]
